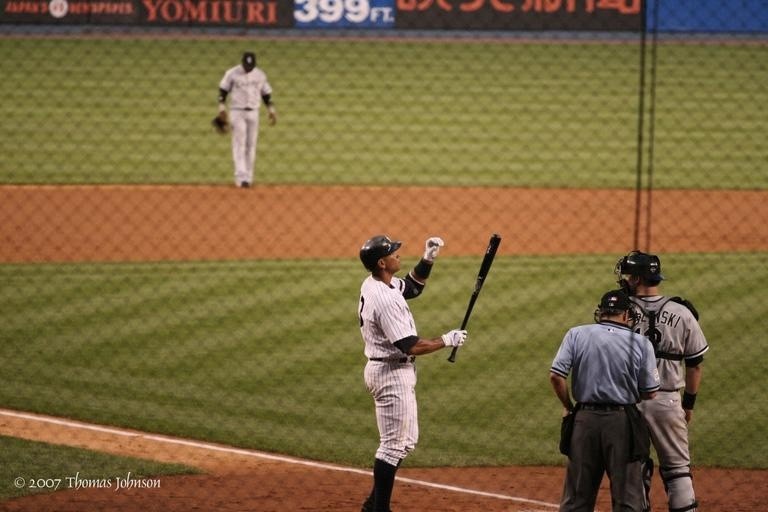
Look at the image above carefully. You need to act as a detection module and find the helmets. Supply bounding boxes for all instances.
[359,236,402,272]
[598,250,663,309]
[243,52,255,72]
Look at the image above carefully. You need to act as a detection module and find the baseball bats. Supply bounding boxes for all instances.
[447,234,501,363]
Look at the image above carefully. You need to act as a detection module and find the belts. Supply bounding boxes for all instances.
[577,403,624,410]
[370,356,416,363]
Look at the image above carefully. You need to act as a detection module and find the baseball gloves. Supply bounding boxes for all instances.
[211,111,230,133]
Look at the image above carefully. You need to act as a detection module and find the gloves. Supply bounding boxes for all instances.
[424,237,444,261]
[441,328,467,347]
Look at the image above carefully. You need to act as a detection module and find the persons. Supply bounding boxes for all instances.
[356,234,468,511]
[616,247,711,511]
[209,49,277,188]
[547,289,660,511]
